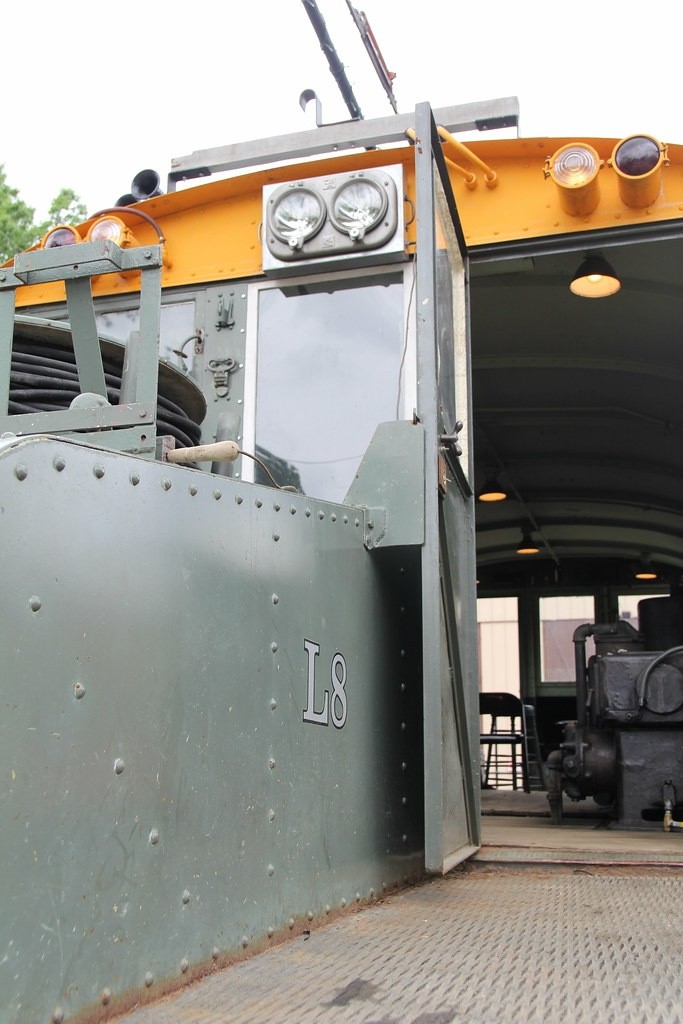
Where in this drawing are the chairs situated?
[479,692,531,795]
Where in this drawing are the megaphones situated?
[114,194,140,208]
[131,169,166,200]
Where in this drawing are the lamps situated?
[634,550,658,580]
[478,458,507,503]
[569,249,621,299]
[514,517,541,555]
